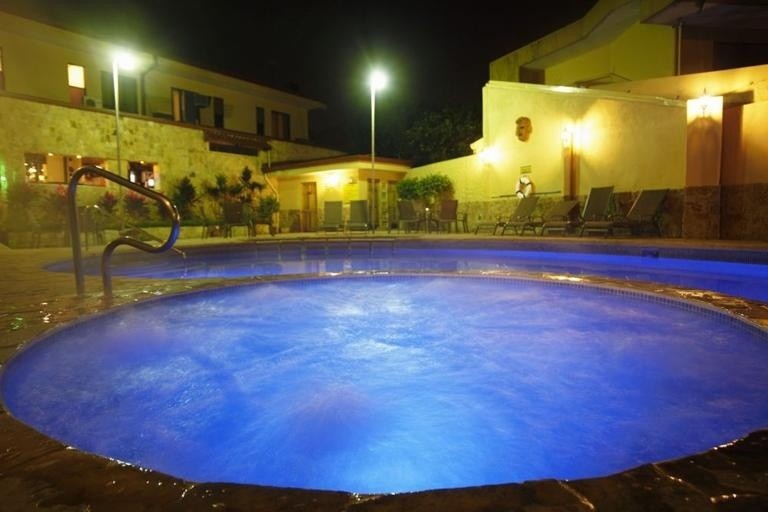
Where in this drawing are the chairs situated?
[493,196,540,235]
[396,199,426,235]
[200,205,228,239]
[250,206,274,236]
[579,188,670,239]
[521,199,580,237]
[346,200,375,237]
[457,202,471,235]
[543,186,613,237]
[320,200,342,235]
[428,199,460,233]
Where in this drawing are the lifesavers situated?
[515,177,533,197]
